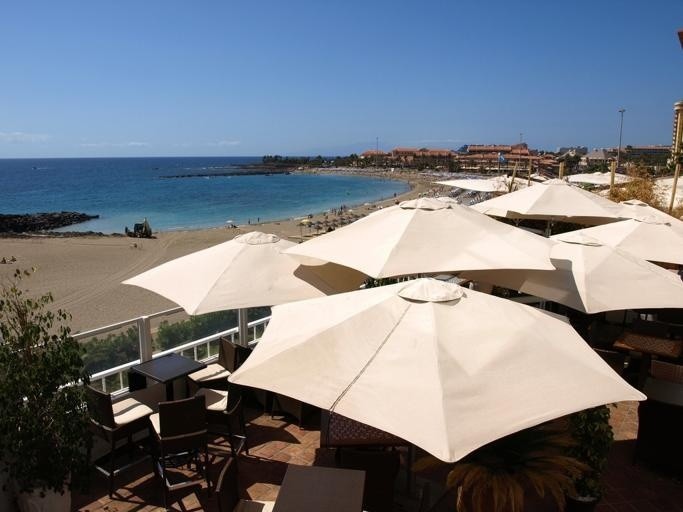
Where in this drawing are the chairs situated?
[79,300,457,512]
[568,308,683,392]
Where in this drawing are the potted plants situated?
[2,252,112,512]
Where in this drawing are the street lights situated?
[617,109,625,168]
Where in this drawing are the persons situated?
[0,257,7,264]
[11,255,16,262]
